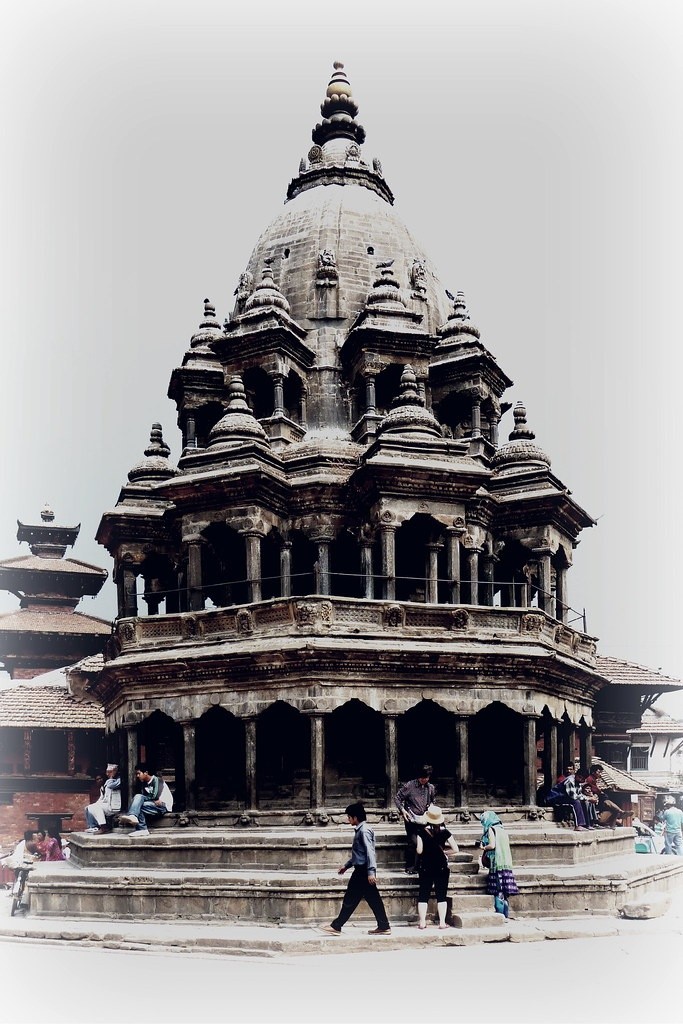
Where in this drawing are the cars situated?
[631,817,678,855]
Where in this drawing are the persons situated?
[652,798,683,856]
[393,765,437,875]
[17,827,71,862]
[318,803,392,935]
[415,806,459,928]
[540,759,634,830]
[479,811,519,923]
[84,763,121,835]
[117,762,173,837]
[90,773,104,804]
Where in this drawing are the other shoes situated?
[84,826,99,832]
[575,825,589,831]
[319,924,341,935]
[593,824,605,829]
[119,815,139,825]
[419,923,427,928]
[128,830,150,837]
[404,868,412,875]
[439,923,450,929]
[94,826,110,834]
[619,811,635,819]
[586,824,595,830]
[368,927,391,934]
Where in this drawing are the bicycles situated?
[0,849,42,916]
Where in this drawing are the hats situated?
[424,806,444,824]
[60,839,70,846]
[107,764,118,771]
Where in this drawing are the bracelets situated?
[484,845,487,850]
